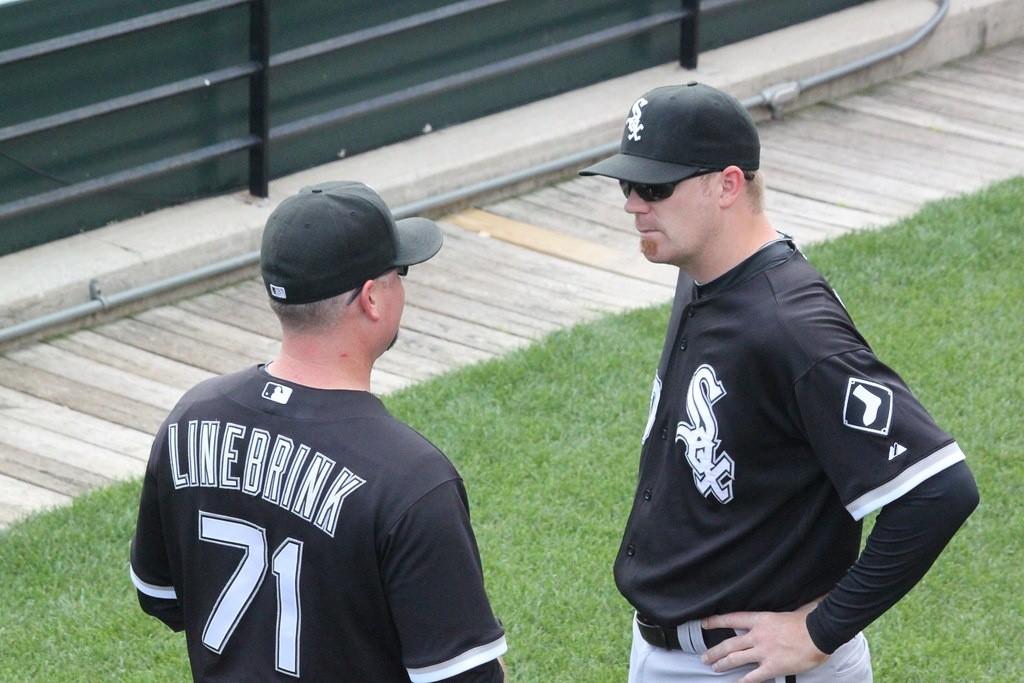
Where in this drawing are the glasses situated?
[347,264,408,305]
[620,168,754,202]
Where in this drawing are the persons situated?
[581,82,979,683]
[130,176,508,683]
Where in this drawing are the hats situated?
[260,180,443,304]
[578,81,760,184]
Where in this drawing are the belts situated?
[635,612,737,652]
